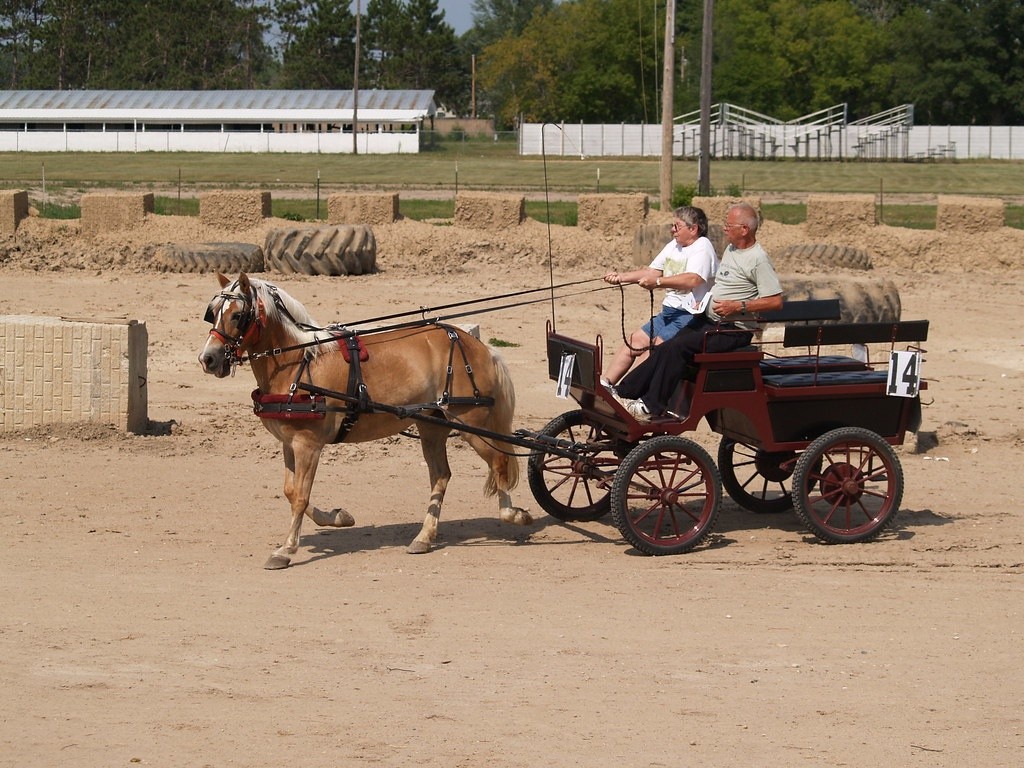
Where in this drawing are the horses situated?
[196,269,532,571]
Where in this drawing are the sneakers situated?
[612,393,653,423]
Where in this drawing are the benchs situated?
[690,317,767,362]
[760,300,875,376]
[762,320,930,398]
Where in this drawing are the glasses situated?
[671,222,685,231]
[723,221,743,229]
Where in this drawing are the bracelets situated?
[741,301,746,315]
[656,277,660,286]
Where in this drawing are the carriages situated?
[195,264,932,570]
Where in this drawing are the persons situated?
[609,204,784,423]
[600,206,719,387]
